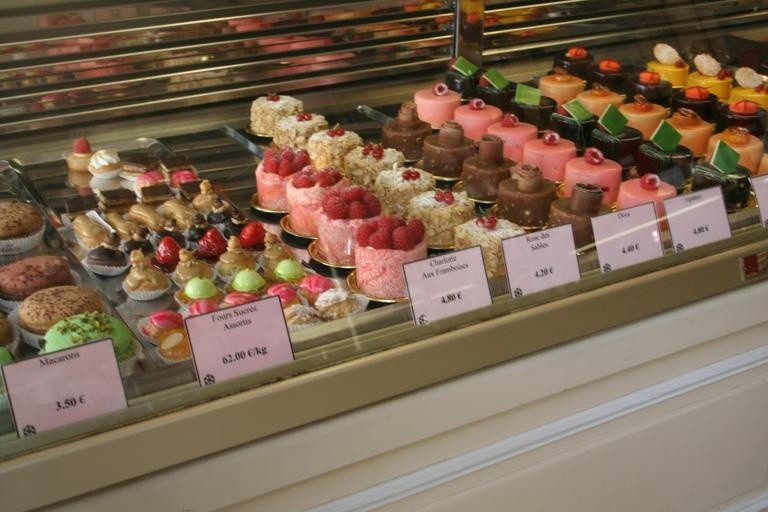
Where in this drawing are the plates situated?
[250,151,582,305]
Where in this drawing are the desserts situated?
[0,44,768,385]
